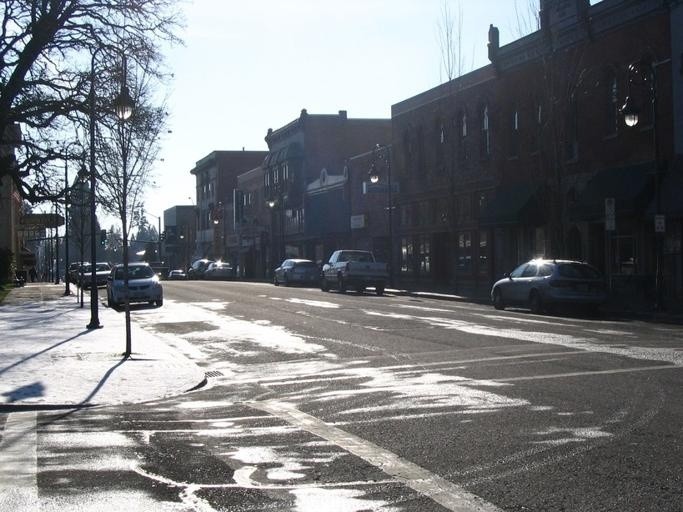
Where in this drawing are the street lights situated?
[177,230,186,263]
[264,192,287,259]
[621,53,666,313]
[87,45,134,330]
[62,142,91,298]
[208,200,227,260]
[368,142,395,288]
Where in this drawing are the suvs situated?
[188,258,216,280]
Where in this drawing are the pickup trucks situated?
[318,250,392,296]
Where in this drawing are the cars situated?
[106,262,163,311]
[67,261,114,290]
[491,259,609,317]
[205,263,233,280]
[168,269,186,280]
[272,257,319,290]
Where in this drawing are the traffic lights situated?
[100,230,105,245]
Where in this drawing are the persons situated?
[28,266,37,282]
